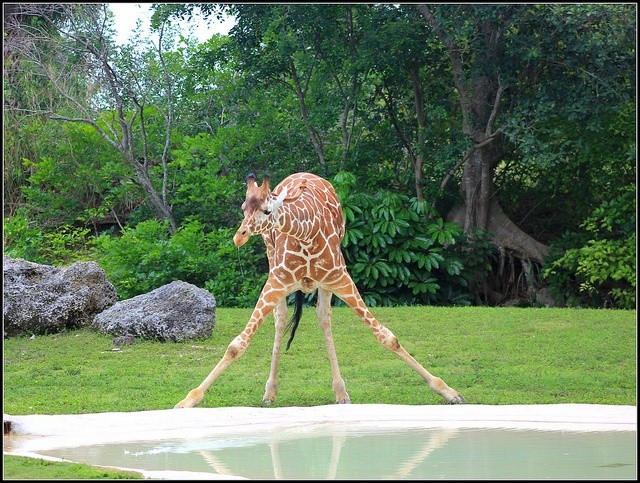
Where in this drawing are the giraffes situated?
[173,172,467,409]
